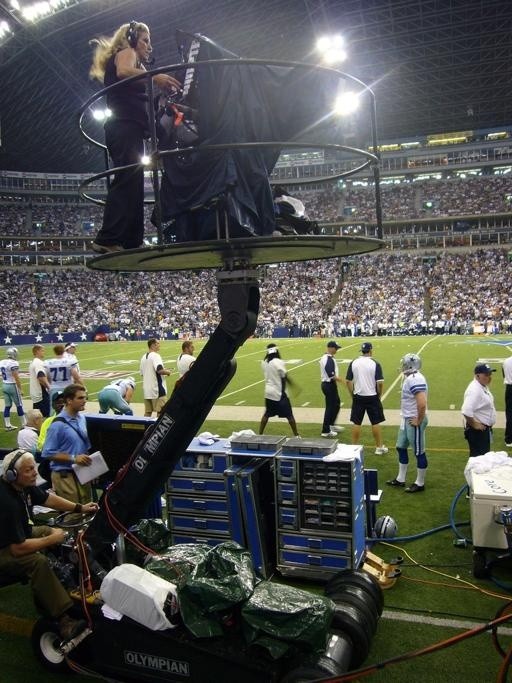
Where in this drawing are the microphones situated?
[145,57,156,65]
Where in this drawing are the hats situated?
[267,346,277,353]
[65,342,78,350]
[360,342,372,351]
[475,363,496,373]
[52,390,63,399]
[328,341,341,347]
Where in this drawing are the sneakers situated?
[330,425,344,431]
[405,483,424,492]
[60,617,85,640]
[321,431,338,436]
[375,444,388,454]
[386,478,406,486]
[5,424,18,430]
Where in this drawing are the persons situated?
[0,449,100,641]
[319,341,346,437]
[1,177,512,342]
[460,364,498,499]
[346,343,389,455]
[386,353,429,493]
[89,20,182,253]
[1,338,197,533]
[501,356,512,447]
[259,343,302,438]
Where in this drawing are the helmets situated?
[6,347,17,359]
[400,353,421,373]
[375,515,397,537]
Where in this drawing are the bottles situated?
[500,504,512,532]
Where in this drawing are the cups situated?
[452,539,467,548]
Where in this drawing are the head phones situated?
[5,449,28,482]
[126,22,138,47]
